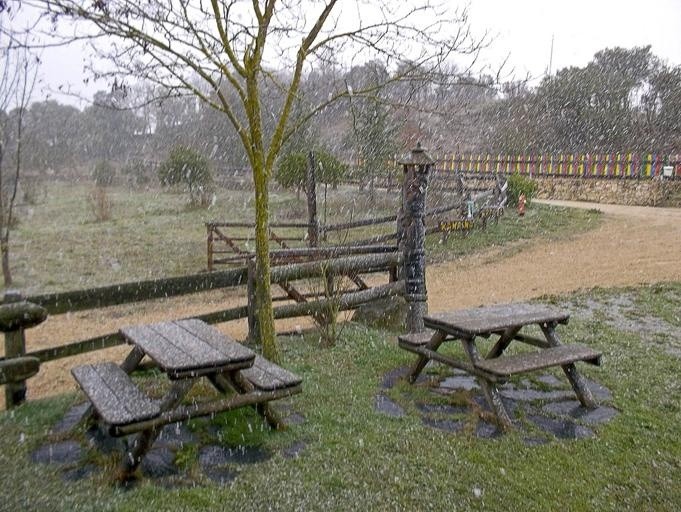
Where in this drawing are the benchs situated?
[474,344,602,378]
[398,331,457,346]
[71,361,160,425]
[240,353,302,391]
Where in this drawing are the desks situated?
[82,318,284,481]
[404,303,599,434]
